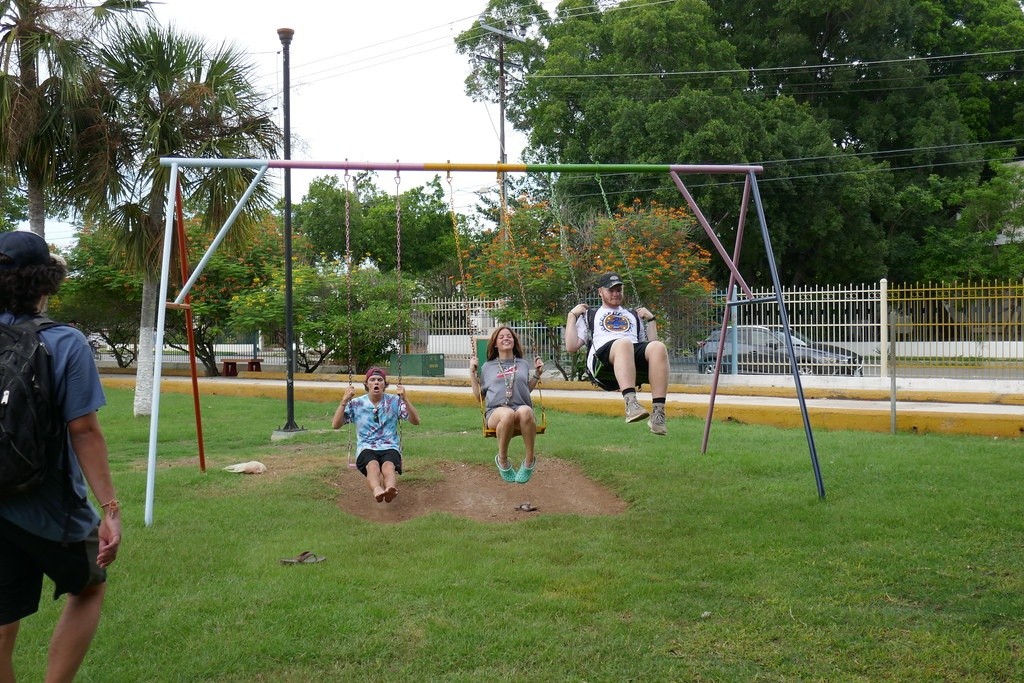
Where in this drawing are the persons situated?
[469,326,545,484]
[565,274,671,435]
[0,231,123,683]
[331,367,420,503]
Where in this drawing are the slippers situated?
[516,455,536,484]
[514,503,537,511]
[280,551,326,564]
[495,454,516,483]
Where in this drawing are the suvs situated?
[697,325,863,376]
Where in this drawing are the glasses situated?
[373,408,379,423]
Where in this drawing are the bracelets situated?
[340,402,347,406]
[100,500,120,518]
[570,311,577,318]
[533,375,540,380]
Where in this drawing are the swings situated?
[445,159,548,438]
[343,157,404,468]
[546,159,651,384]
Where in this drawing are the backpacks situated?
[0,320,69,499]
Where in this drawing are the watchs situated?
[648,316,656,322]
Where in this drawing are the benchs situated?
[220,359,263,377]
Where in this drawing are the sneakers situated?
[648,412,666,435]
[625,398,650,423]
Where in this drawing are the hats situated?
[597,272,624,289]
[365,367,386,392]
[0,231,66,272]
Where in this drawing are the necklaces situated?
[496,356,516,404]
